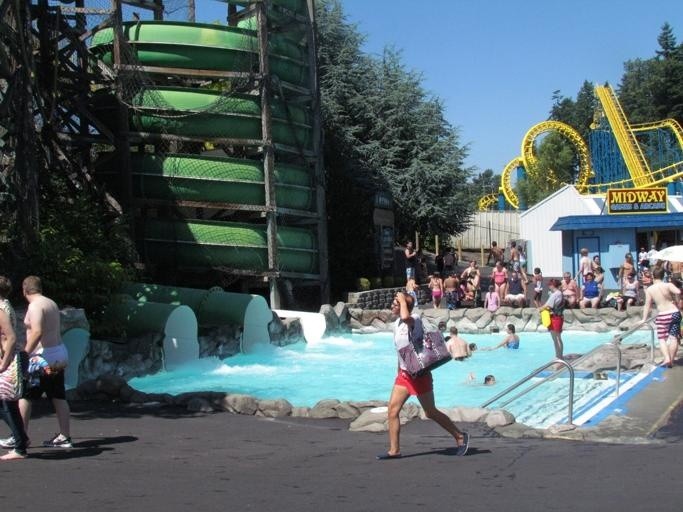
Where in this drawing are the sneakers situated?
[375,452,401,460]
[40,432,73,448]
[456,432,470,458]
[0,436,32,460]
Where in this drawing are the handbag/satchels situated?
[0,352,25,402]
[398,320,453,379]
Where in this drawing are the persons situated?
[0,275,30,462]
[374,291,469,463]
[0,274,75,451]
[403,238,682,389]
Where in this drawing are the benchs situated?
[458,299,530,307]
